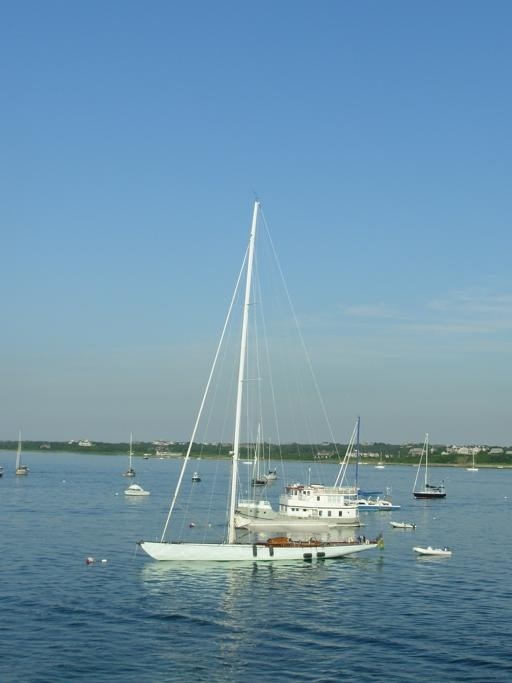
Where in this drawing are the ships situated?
[235,462,368,543]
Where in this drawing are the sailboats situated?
[251,416,400,511]
[135,199,384,561]
[122,432,135,476]
[466,446,480,471]
[139,558,359,671]
[15,428,31,474]
[411,431,447,499]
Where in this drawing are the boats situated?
[0,466,4,476]
[390,521,417,527]
[123,483,150,496]
[413,546,452,554]
[496,465,503,468]
[142,456,148,458]
[191,471,201,480]
